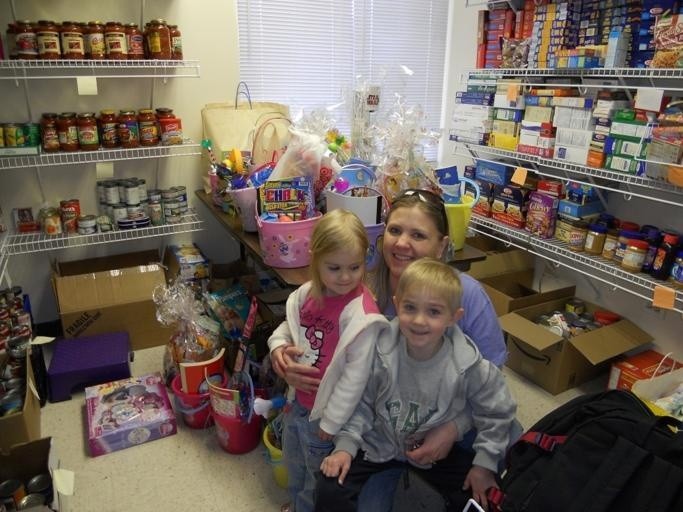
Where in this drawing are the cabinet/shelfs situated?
[447,0,682,317]
[1,60,203,292]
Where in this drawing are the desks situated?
[194,186,487,290]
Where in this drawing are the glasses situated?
[390,189,449,235]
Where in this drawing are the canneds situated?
[6,17,183,62]
[0,287,33,418]
[0,473,55,512]
[539,299,603,338]
[1,108,183,150]
[37,178,187,235]
[567,213,683,290]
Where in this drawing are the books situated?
[178,346,225,393]
[202,365,240,418]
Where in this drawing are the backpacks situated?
[486,389,682,511]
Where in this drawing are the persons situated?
[308,255,524,511]
[281,186,510,511]
[265,206,388,511]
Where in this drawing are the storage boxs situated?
[0,345,60,512]
[465,231,655,397]
[49,248,178,351]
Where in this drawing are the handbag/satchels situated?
[630,366,682,431]
[201,81,288,195]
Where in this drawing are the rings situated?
[428,459,437,465]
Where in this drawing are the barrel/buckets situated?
[259,422,290,490]
[208,406,263,455]
[442,176,481,253]
[210,152,391,272]
[171,371,228,428]
